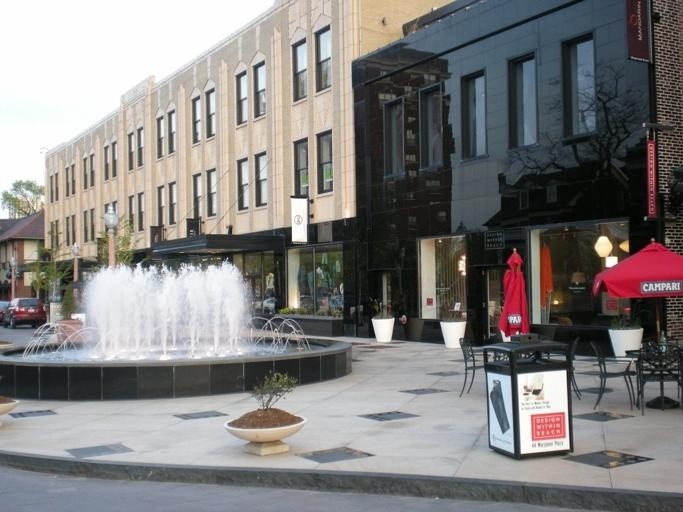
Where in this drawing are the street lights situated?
[9,255,16,303]
[104,197,122,269]
[71,240,81,314]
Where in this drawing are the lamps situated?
[571,271,586,287]
[594,223,629,268]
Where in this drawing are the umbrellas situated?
[497,247,530,337]
[539,241,554,324]
[590,238,683,398]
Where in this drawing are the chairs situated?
[458,335,683,414]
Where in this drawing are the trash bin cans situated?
[483,334,574,459]
[48,301,68,325]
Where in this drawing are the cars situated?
[0,302,10,321]
[249,292,344,318]
[2,297,48,329]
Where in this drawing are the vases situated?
[0,394,20,428]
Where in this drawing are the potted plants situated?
[608,309,644,361]
[223,369,308,456]
[440,301,466,349]
[370,302,395,342]
